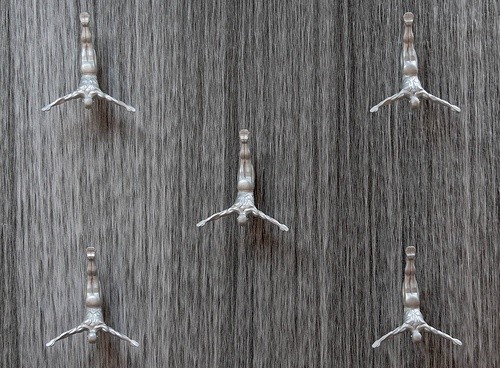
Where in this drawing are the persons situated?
[46,248,140,352]
[369,12,464,119]
[40,12,134,113]
[193,127,291,235]
[369,245,464,346]
